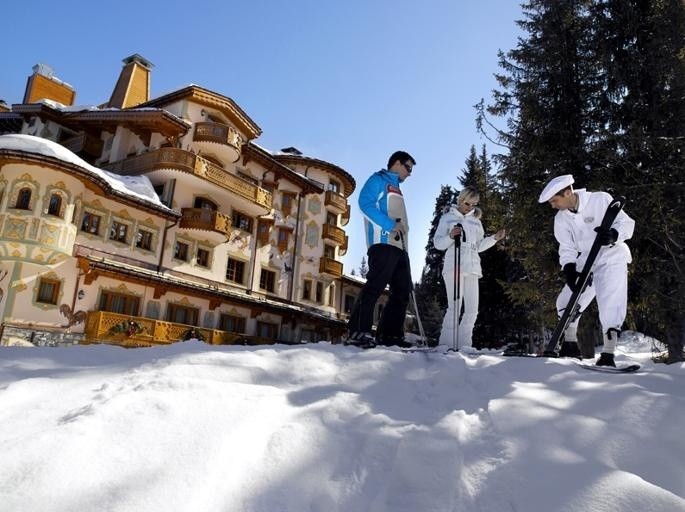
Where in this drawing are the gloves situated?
[594,227,616,246]
[564,263,585,293]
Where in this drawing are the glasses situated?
[403,162,412,173]
[464,200,477,207]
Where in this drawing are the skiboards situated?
[443,345,508,356]
[548,194,640,373]
[344,343,450,353]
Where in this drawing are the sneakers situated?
[596,353,615,366]
[376,334,412,347]
[342,331,377,348]
[558,342,583,359]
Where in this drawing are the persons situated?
[537,174,636,368]
[343,151,417,349]
[431,186,506,353]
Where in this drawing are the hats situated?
[538,175,575,203]
